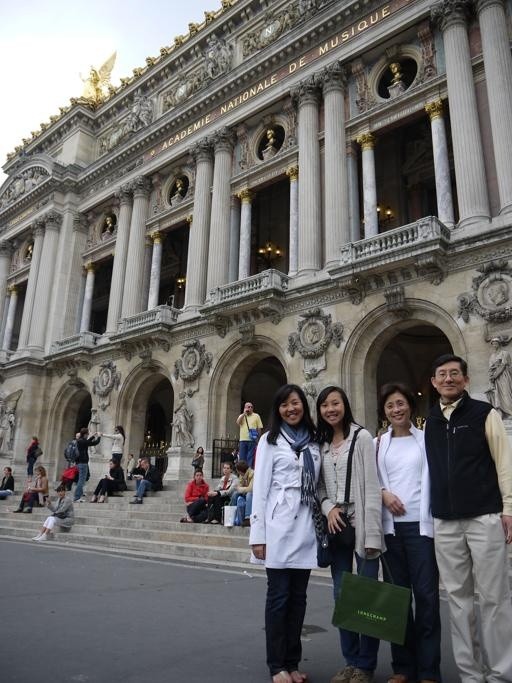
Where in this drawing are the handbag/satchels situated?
[318,511,355,567]
[33,448,42,458]
[54,512,66,518]
[249,429,259,440]
[23,493,31,501]
[223,506,238,527]
[192,458,200,466]
[332,570,411,646]
[60,467,79,479]
[206,503,221,522]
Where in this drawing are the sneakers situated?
[180,518,188,522]
[32,532,48,541]
[13,507,32,513]
[74,496,105,503]
[330,666,373,682]
[242,518,250,527]
[210,519,219,524]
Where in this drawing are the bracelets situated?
[241,414,244,416]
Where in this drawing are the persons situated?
[25,436,40,483]
[302,371,319,423]
[184,447,254,526]
[372,383,441,683]
[424,354,512,683]
[31,485,74,541]
[0,405,15,451]
[0,467,14,496]
[55,426,163,504]
[236,401,264,469]
[13,465,49,513]
[316,387,387,683]
[85,407,102,452]
[484,338,512,416]
[248,383,323,683]
[173,389,199,446]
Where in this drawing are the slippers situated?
[273,670,310,682]
[387,675,433,682]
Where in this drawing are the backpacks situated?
[64,439,80,460]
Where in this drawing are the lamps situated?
[258,186,282,268]
[361,203,395,234]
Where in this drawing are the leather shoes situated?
[134,493,147,497]
[129,499,142,504]
